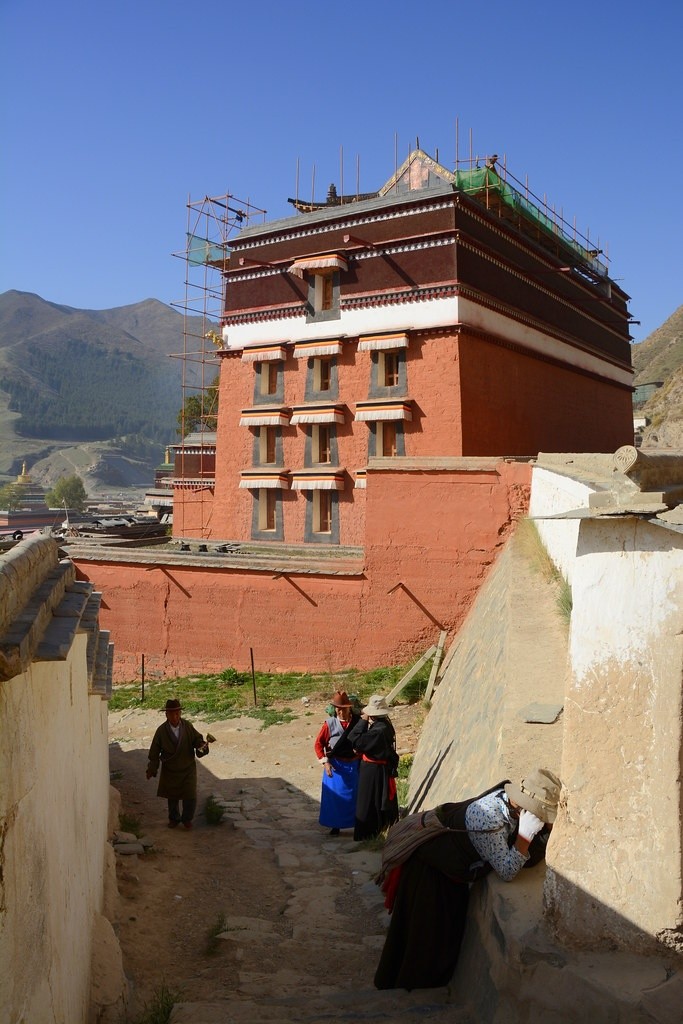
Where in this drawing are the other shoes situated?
[180,818,192,830]
[329,828,340,836]
[167,816,180,828]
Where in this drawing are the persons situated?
[145,699,209,830]
[315,691,362,835]
[374,769,562,992]
[347,695,400,841]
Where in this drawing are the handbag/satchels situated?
[374,809,446,882]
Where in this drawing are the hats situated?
[504,769,561,823]
[328,691,354,707]
[160,699,184,711]
[361,694,389,716]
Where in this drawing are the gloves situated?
[518,811,544,842]
